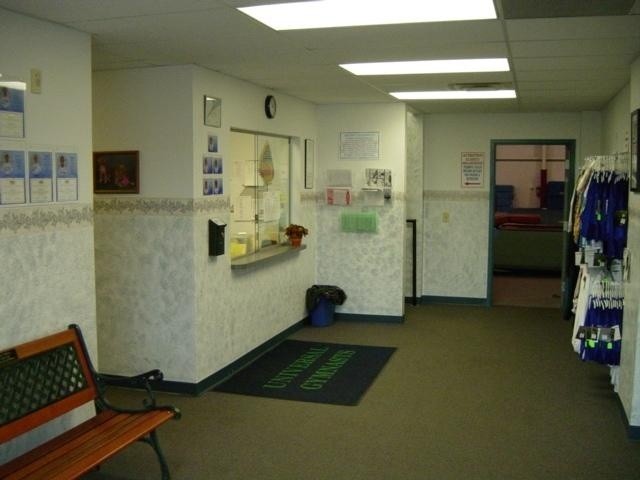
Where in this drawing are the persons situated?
[2,154,14,176]
[203,180,210,195]
[1,87,12,108]
[203,158,209,173]
[57,155,70,175]
[30,154,42,176]
[214,180,220,194]
[213,159,220,174]
[208,136,215,152]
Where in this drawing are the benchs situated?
[0,323,183,479]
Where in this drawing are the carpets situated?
[209,338,398,408]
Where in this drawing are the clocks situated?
[265,93,278,120]
[203,94,222,129]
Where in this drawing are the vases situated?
[289,239,303,247]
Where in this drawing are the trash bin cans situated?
[309,285,335,326]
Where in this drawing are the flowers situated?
[285,223,309,239]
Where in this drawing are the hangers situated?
[588,281,626,310]
[581,154,629,183]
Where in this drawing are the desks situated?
[491,208,562,279]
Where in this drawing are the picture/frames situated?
[629,107,639,196]
[92,149,143,197]
[303,137,317,190]
[325,188,353,207]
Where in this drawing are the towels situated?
[341,213,378,233]
